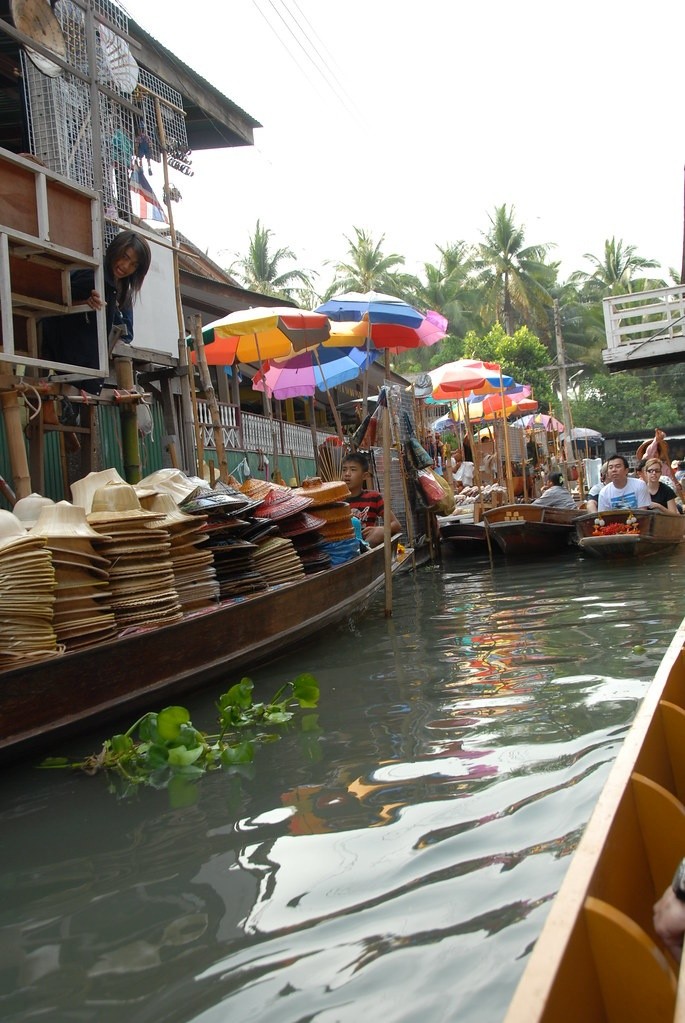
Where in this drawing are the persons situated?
[427,436,489,493]
[37,230,151,450]
[586,455,651,513]
[341,452,401,549]
[531,472,578,510]
[636,432,685,515]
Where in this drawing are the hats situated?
[636,439,669,462]
[670,460,679,468]
[0,468,356,670]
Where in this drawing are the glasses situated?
[646,469,661,473]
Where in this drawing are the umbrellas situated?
[187,306,331,483]
[252,291,448,482]
[556,427,606,459]
[510,413,564,461]
[420,357,539,464]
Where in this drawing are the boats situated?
[1,530,416,755]
[426,372,588,561]
[505,618,685,1023]
[571,507,685,562]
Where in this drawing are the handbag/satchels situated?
[403,412,455,517]
[348,389,388,451]
